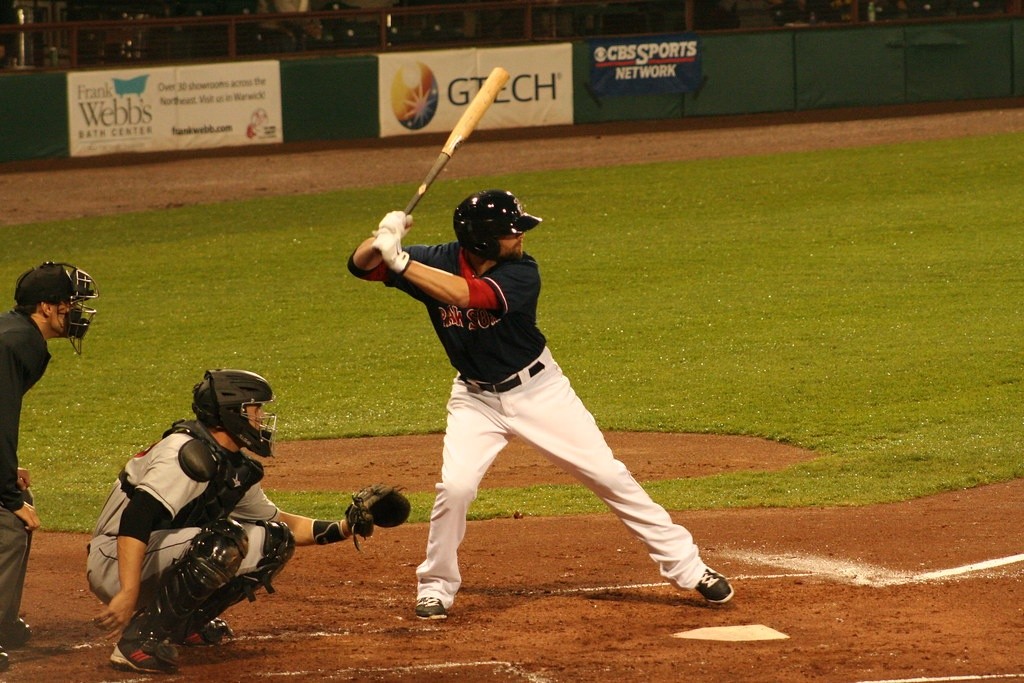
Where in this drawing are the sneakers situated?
[415,597,448,619]
[110,607,182,673]
[169,621,207,645]
[694,567,735,603]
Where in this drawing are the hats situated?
[14,261,95,303]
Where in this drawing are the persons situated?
[347,189,735,620]
[87,368,411,672]
[0,261,98,672]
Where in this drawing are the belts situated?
[87,534,113,555]
[459,361,544,393]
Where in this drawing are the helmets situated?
[453,189,542,260]
[191,368,276,458]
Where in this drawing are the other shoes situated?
[0,646,8,666]
[0,619,33,645]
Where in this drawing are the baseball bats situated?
[372,66,512,258]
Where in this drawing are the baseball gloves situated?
[344,479,414,552]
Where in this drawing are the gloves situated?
[371,210,412,273]
